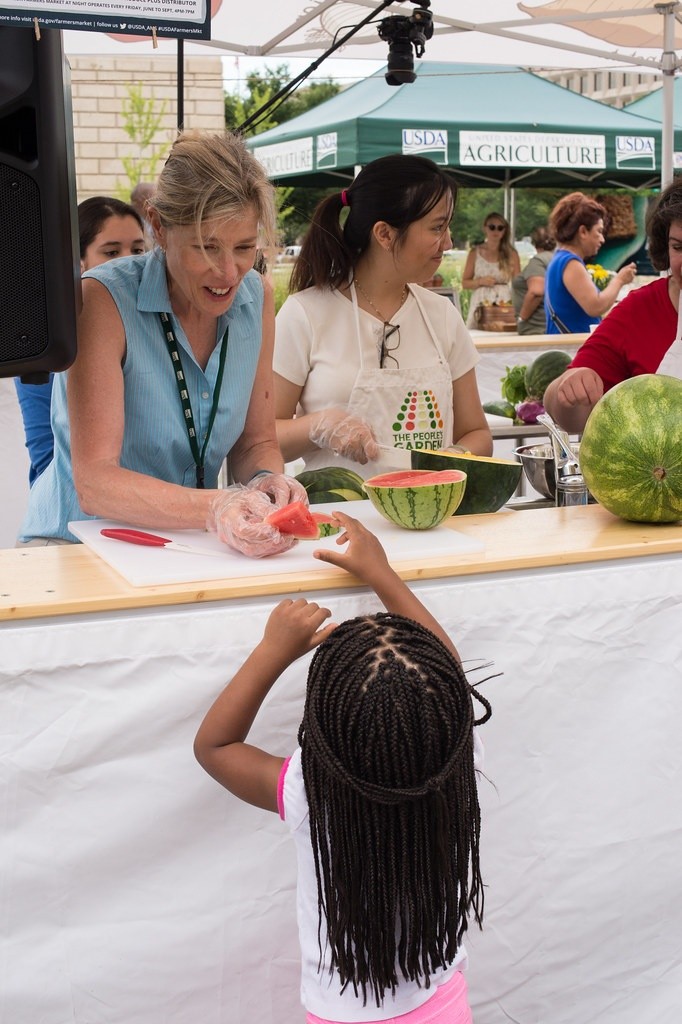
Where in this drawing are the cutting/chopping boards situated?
[68,500,487,587]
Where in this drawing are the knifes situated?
[100,528,238,561]
[364,445,411,468]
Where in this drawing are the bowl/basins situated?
[513,442,594,501]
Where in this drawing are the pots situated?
[474,305,516,330]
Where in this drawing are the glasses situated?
[486,224,507,231]
[380,322,400,369]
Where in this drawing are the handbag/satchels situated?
[474,303,517,332]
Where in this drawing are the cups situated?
[551,433,587,507]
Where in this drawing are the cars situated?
[276,245,302,263]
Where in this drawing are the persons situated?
[543,192,637,333]
[543,182,682,433]
[462,212,521,326]
[130,183,155,247]
[14,198,145,485]
[511,227,556,334]
[273,154,494,481]
[16,134,311,557]
[193,510,493,1024]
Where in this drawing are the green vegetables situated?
[500,365,528,404]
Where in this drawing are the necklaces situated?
[354,278,406,320]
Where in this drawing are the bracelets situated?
[518,316,523,321]
[252,470,273,478]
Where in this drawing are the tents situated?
[104,1,682,276]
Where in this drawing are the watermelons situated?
[482,400,516,419]
[411,448,522,516]
[364,469,467,530]
[309,512,341,537]
[294,466,368,504]
[579,374,682,522]
[264,500,321,541]
[524,351,572,400]
